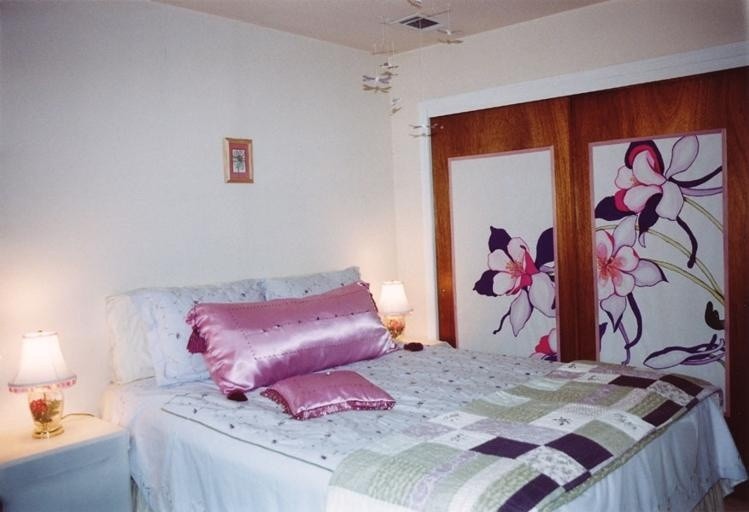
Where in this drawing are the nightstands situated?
[1,415,130,512]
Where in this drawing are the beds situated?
[100,346,724,512]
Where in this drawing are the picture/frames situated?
[225,138,254,184]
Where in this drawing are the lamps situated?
[8,330,77,440]
[376,280,413,345]
